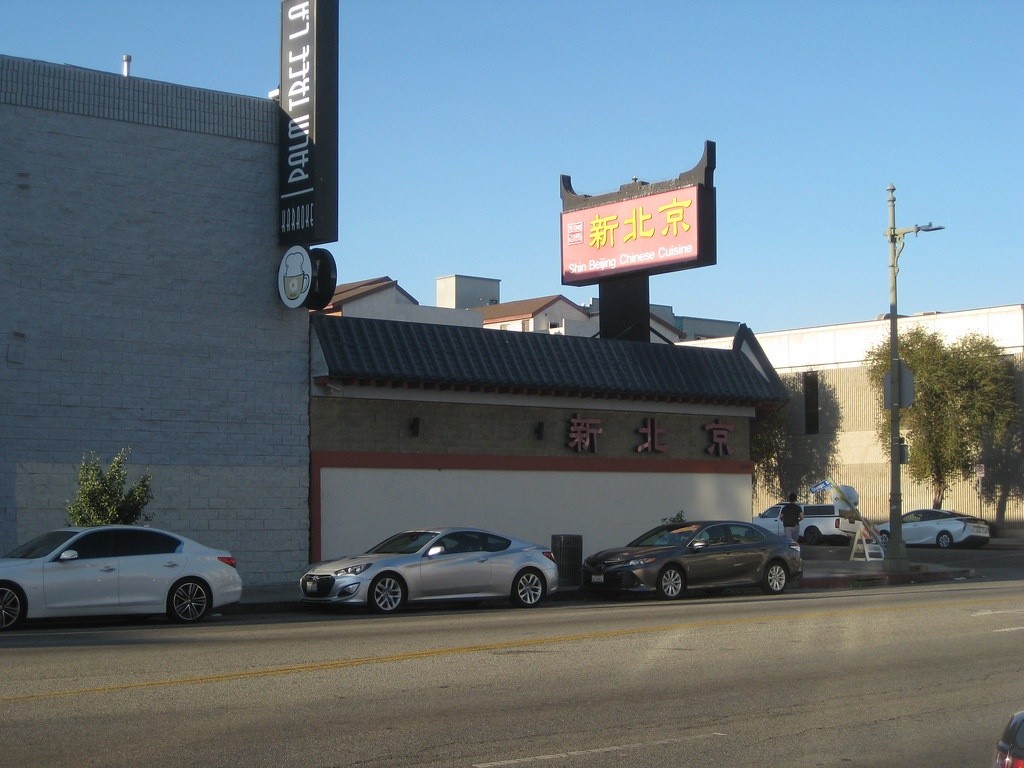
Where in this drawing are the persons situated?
[780,493,804,542]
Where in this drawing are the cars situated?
[579,520,805,601]
[0,524,244,632]
[872,508,990,550]
[298,526,560,615]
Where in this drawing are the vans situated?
[753,501,871,547]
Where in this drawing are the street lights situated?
[883,180,946,576]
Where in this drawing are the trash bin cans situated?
[551,534,583,587]
